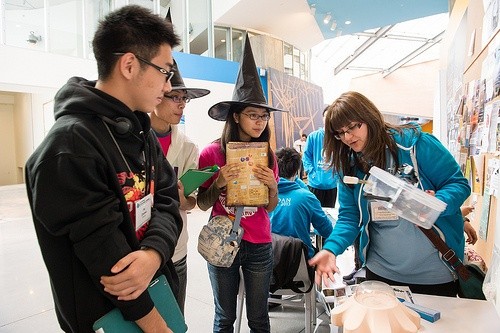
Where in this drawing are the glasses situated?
[164,94,192,104]
[333,121,364,140]
[111,52,175,83]
[240,111,271,122]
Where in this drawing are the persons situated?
[293,134,307,175]
[24,4,183,333]
[302,106,340,208]
[459,205,478,245]
[148,7,210,319]
[267,147,334,267]
[196,32,278,333]
[308,91,471,297]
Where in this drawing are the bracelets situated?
[463,218,470,222]
[269,189,279,198]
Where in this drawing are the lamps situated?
[310,5,337,30]
[28,30,42,46]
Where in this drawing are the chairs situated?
[231,229,325,333]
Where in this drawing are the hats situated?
[169,57,211,100]
[208,33,290,121]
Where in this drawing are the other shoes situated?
[267,296,283,309]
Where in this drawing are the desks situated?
[343,293,500,333]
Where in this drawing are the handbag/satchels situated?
[197,214,244,269]
[456,243,489,301]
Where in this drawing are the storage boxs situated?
[361,166,448,230]
